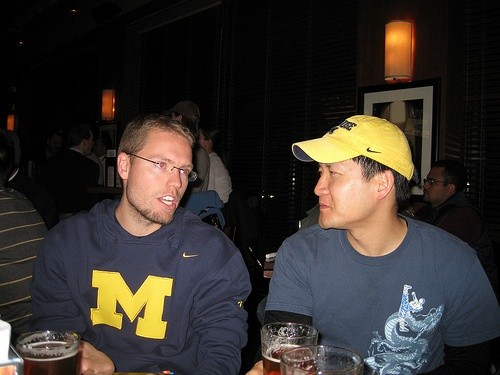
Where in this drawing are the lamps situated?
[384,17,416,81]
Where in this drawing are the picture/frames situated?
[357,77,439,203]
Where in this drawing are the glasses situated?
[126,152,198,182]
[424,178,454,185]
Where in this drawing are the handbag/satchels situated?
[185,189,226,231]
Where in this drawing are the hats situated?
[291,114,414,181]
[170,100,201,122]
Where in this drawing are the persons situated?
[0,124,112,346]
[244,115,500,375]
[32,114,253,375]
[163,101,500,305]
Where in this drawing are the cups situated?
[280,345,364,375]
[260,322,319,375]
[16,329,81,375]
[0,320,12,362]
